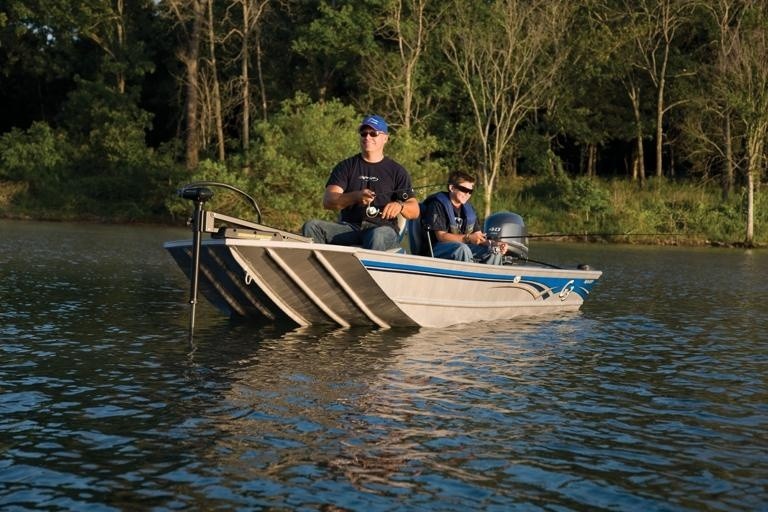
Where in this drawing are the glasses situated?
[360,131,384,138]
[455,184,474,194]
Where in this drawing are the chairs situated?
[388,213,407,253]
[409,202,442,256]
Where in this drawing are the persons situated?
[417,167,510,268]
[300,111,423,254]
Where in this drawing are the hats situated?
[357,115,389,134]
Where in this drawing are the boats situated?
[162,182,603,331]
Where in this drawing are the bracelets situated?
[395,199,405,215]
[465,232,472,244]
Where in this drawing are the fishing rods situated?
[364,181,466,219]
[500,254,563,269]
[484,233,704,243]
